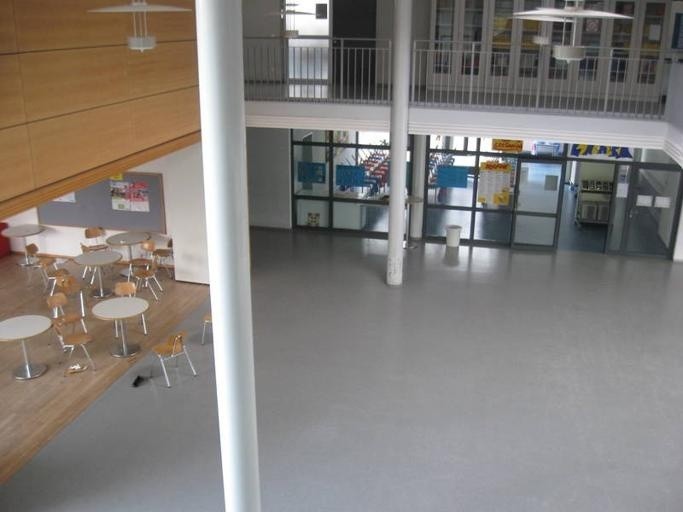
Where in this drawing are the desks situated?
[0,315,53,380]
[1,224,45,266]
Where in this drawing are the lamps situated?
[264,0,315,39]
[88,0,192,50]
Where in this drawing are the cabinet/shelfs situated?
[578,192,611,223]
[425,0,671,104]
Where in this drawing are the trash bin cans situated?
[445,224,462,248]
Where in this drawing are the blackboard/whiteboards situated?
[37,172,167,235]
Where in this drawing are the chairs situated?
[25,227,212,388]
